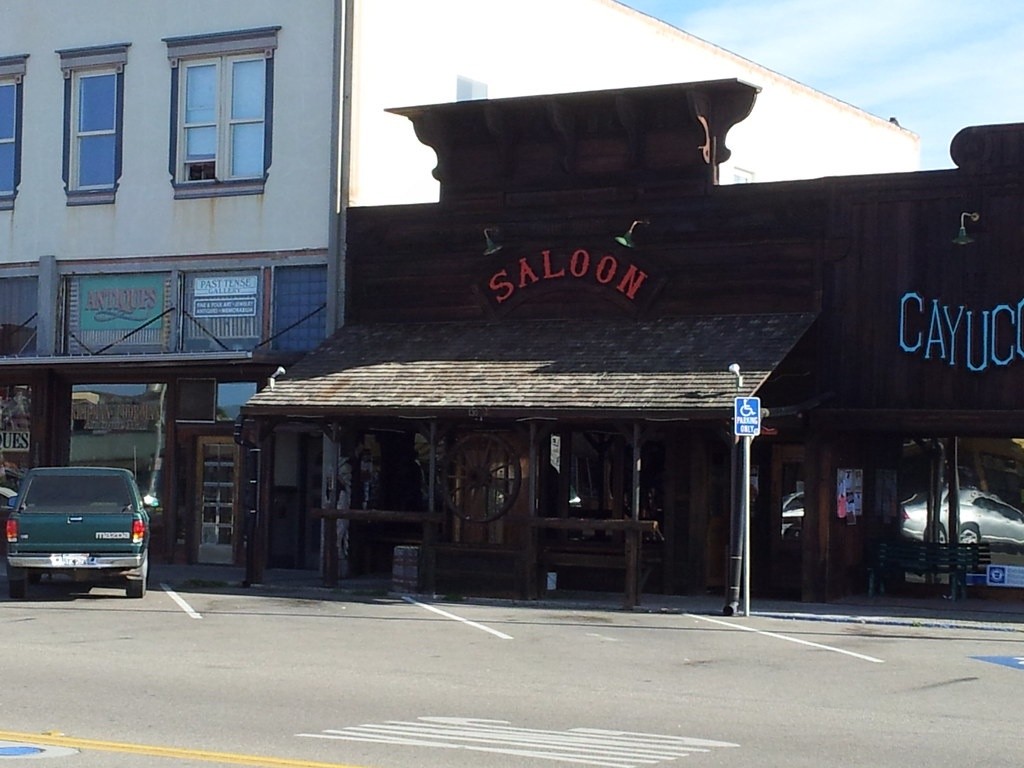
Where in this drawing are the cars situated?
[779,489,807,540]
[897,487,1024,548]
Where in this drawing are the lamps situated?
[481,227,503,255]
[951,211,980,244]
[616,219,651,247]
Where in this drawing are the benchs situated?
[866,539,993,606]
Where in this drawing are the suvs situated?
[5,467,150,599]
